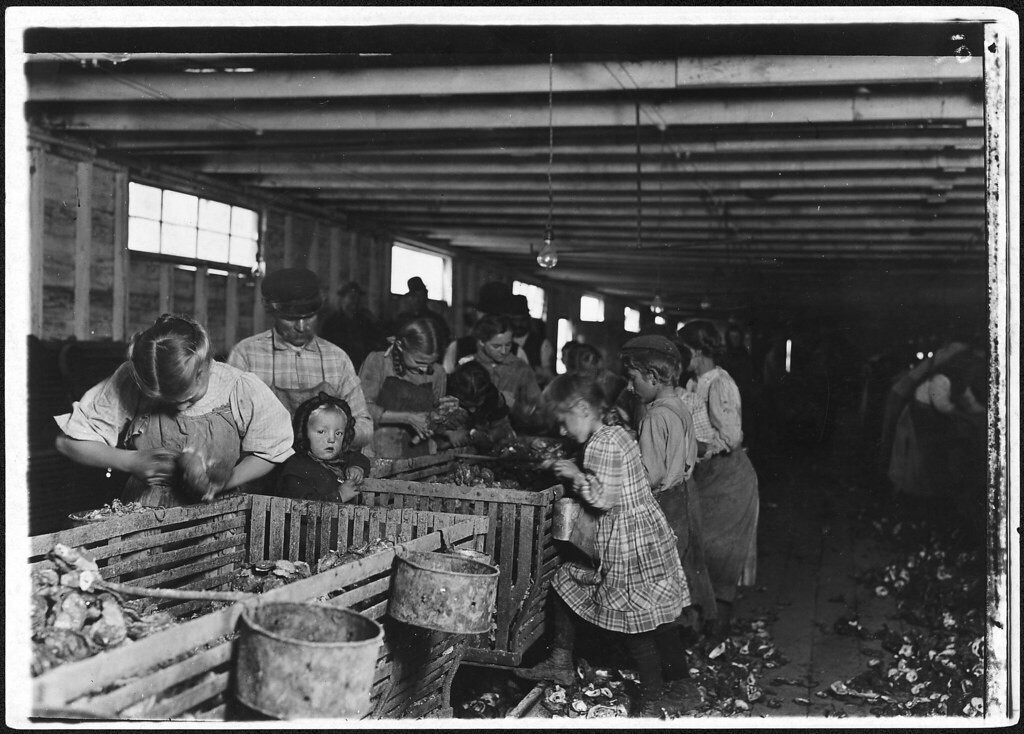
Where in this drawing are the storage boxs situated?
[31,493,490,720]
[356,443,564,667]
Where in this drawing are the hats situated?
[473,281,512,312]
[407,277,424,288]
[508,295,529,314]
[261,268,324,317]
[622,335,685,362]
[337,282,365,296]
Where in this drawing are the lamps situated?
[535,51,561,271]
[651,133,666,315]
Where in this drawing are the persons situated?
[610,334,719,713]
[397,277,556,432]
[319,281,377,377]
[435,360,509,448]
[227,268,373,494]
[358,318,459,458]
[676,317,757,640]
[276,391,370,502]
[52,313,295,589]
[513,370,693,720]
[542,342,626,419]
[881,342,983,502]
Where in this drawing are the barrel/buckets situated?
[389,550,501,636]
[232,600,386,720]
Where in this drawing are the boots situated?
[514,647,575,687]
[631,647,666,701]
